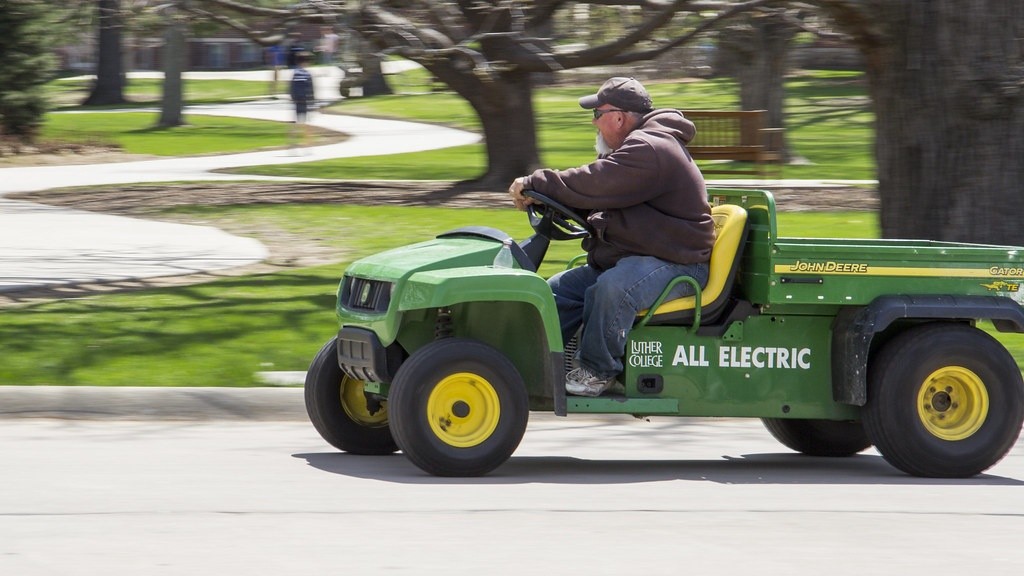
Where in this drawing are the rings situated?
[510,189,514,192]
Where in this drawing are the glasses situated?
[594,108,628,118]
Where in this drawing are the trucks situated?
[304,186,1024,477]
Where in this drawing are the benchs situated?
[682,110,787,177]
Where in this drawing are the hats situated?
[579,77,651,112]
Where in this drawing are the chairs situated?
[633,203,750,328]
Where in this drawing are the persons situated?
[509,76,715,396]
[287,24,339,124]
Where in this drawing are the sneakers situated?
[566,368,616,398]
